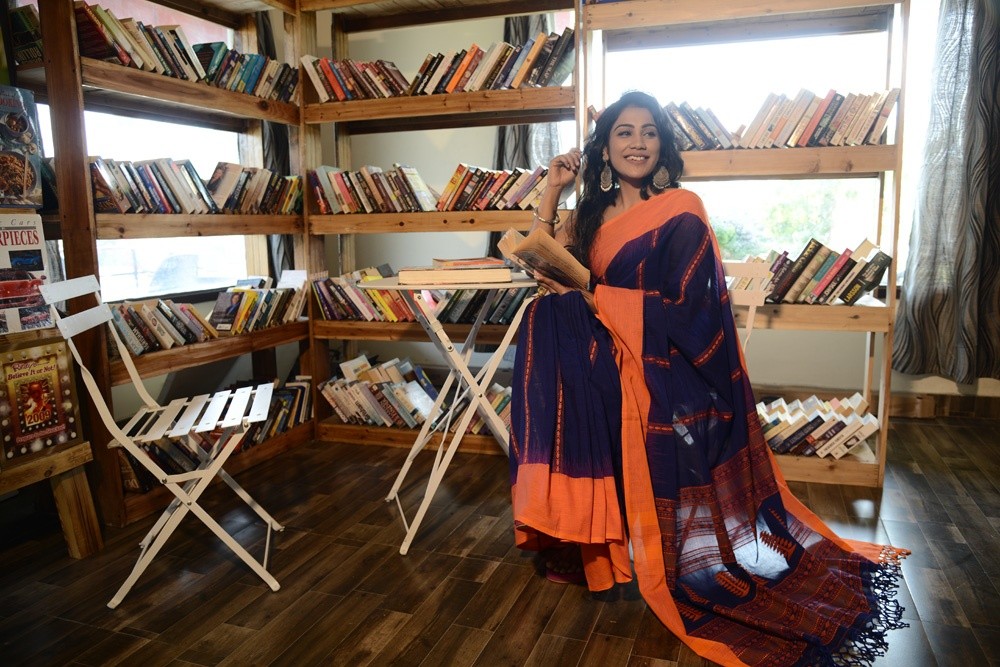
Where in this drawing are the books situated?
[498,227,593,291]
[41,155,303,215]
[725,236,893,307]
[115,376,314,492]
[299,27,577,104]
[0,339,83,470]
[0,212,57,334]
[587,88,901,150]
[756,391,880,460]
[317,355,512,435]
[312,256,545,325]
[311,161,578,216]
[0,86,46,210]
[103,269,309,358]
[2,0,300,105]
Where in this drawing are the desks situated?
[354,270,555,564]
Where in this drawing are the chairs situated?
[31,272,288,608]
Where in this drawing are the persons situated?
[509,90,718,584]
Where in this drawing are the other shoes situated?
[545,548,587,584]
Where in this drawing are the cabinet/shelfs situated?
[12,0,312,529]
[308,0,589,452]
[580,0,912,519]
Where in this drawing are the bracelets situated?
[532,206,562,224]
